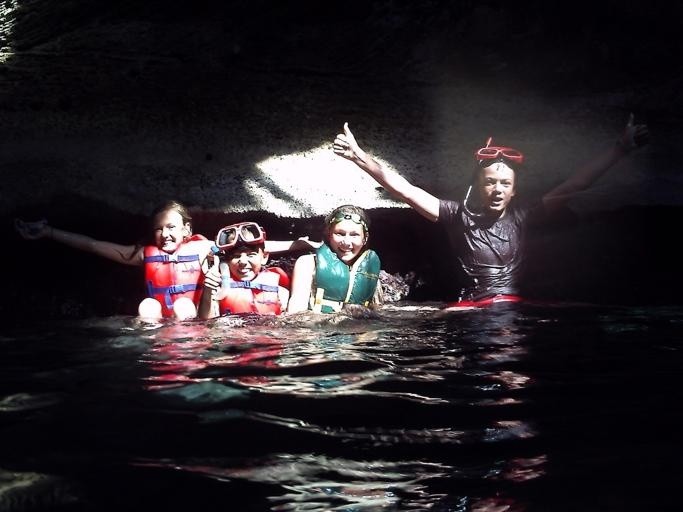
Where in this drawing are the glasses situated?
[215,222,266,250]
[330,213,369,232]
[475,146,523,164]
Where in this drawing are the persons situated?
[191,218,294,324]
[11,198,325,326]
[332,112,650,305]
[282,204,382,324]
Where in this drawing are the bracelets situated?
[44,226,66,251]
